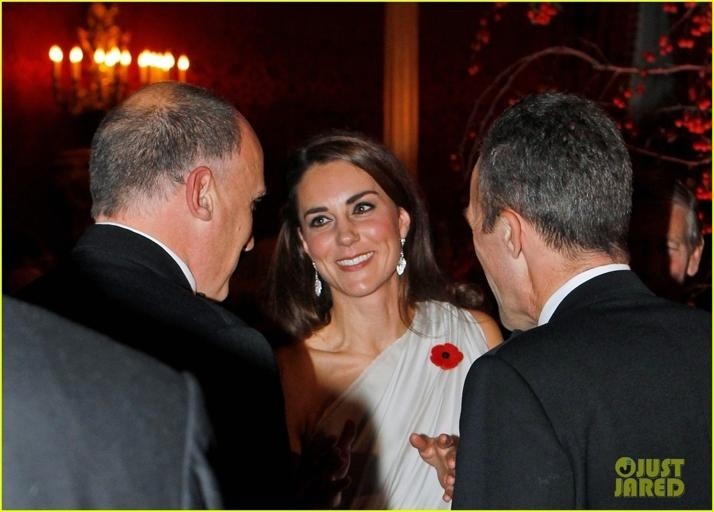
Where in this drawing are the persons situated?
[627,165,704,303]
[19,82,287,512]
[451,91,710,508]
[269,136,503,512]
